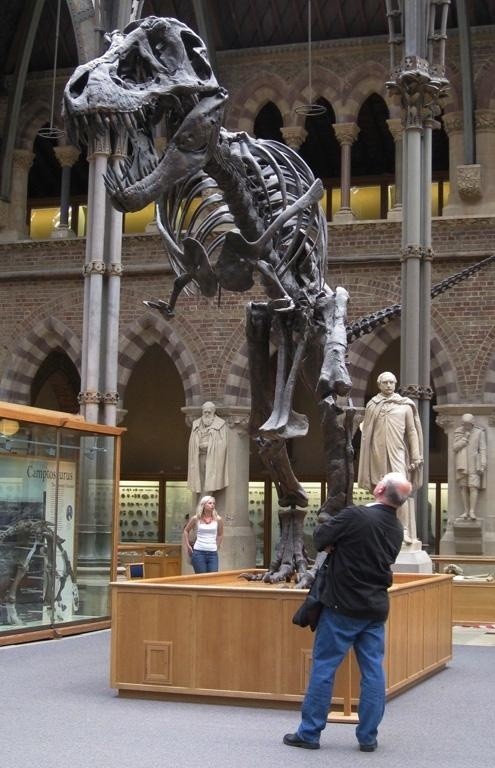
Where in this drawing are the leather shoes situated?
[360,739,378,751]
[283,733,320,750]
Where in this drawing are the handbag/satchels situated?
[292,596,322,632]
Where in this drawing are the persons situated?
[183,494,223,573]
[67,505,72,520]
[187,400,229,494]
[451,412,486,520]
[280,472,413,752]
[358,370,426,545]
